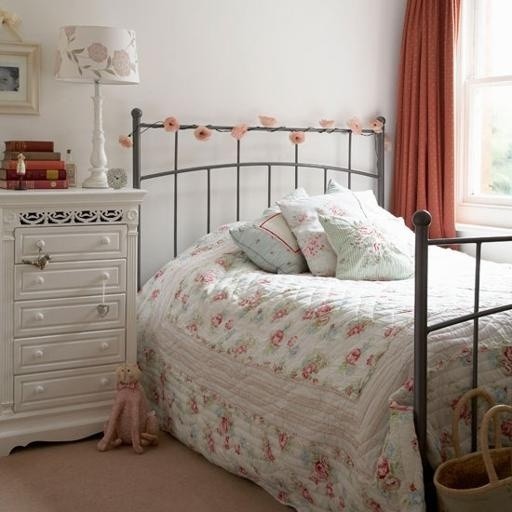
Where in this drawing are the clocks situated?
[107,168,128,189]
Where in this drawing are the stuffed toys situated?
[98,363,158,455]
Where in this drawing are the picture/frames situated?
[0,40,41,114]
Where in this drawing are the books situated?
[0,140,68,190]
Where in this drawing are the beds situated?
[131,108,512,512]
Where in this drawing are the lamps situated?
[52,23,139,189]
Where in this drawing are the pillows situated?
[228,178,418,281]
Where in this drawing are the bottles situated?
[64,148,77,187]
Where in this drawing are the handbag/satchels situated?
[432,389,511,512]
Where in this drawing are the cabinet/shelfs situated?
[0,187,148,458]
